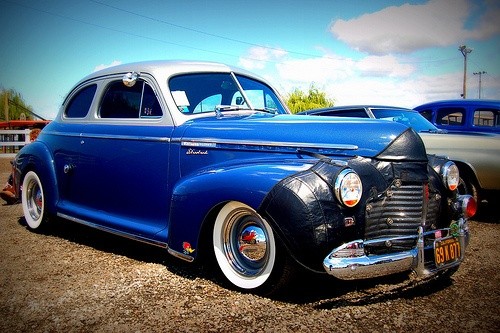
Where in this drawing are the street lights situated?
[459,45,472,100]
[473,70,486,99]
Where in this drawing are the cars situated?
[7,60,477,297]
[412,100,500,138]
[294,105,500,219]
